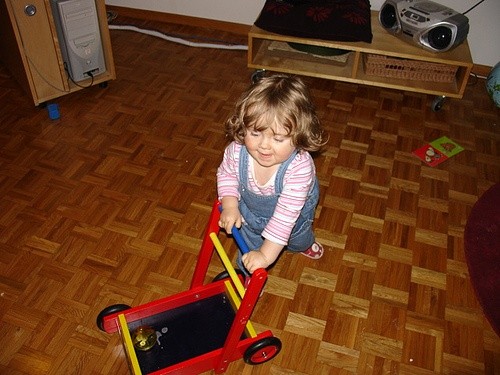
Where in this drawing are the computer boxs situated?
[57,0,106,82]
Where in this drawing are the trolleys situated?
[94,200,282,375]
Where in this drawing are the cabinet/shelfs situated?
[0,0,117,108]
[247,8,473,109]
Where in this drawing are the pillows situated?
[253,0,372,44]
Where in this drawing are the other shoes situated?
[245,276,264,297]
[300,241,325,259]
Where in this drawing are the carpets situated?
[464,182,500,338]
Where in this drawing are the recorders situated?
[378,0,485,52]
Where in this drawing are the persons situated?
[216,75,330,296]
